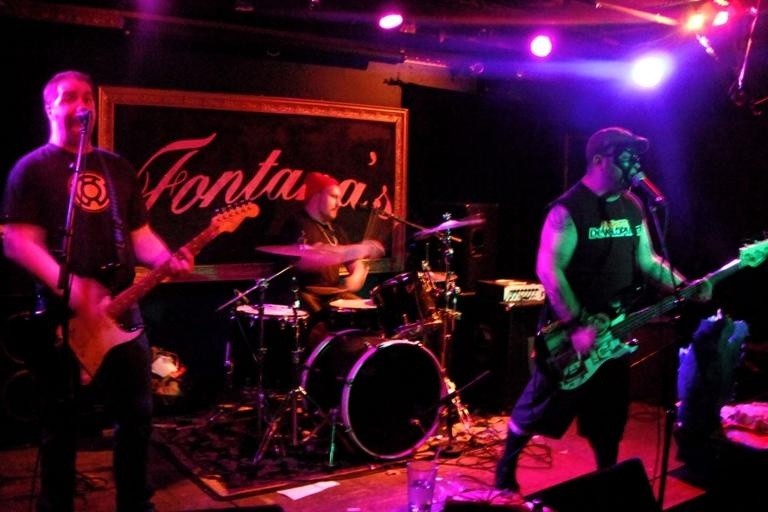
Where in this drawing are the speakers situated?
[508,456,664,511]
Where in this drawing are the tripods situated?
[215,271,358,465]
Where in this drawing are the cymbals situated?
[254,246,346,261]
[414,221,484,235]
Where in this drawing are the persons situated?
[260,170,386,351]
[493,126,714,494]
[3,70,198,511]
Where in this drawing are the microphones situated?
[76,108,92,123]
[628,167,667,208]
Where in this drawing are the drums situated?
[233,303,312,392]
[371,273,443,341]
[299,327,448,461]
[328,296,384,337]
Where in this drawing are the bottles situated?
[406,462,436,512]
[532,498,543,511]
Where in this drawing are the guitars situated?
[54,196,261,383]
[534,239,768,394]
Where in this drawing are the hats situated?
[305,172,340,205]
[586,127,649,158]
